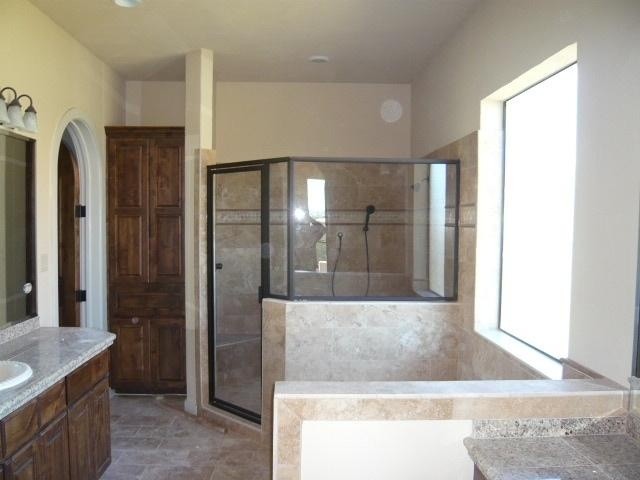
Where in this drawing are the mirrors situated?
[1,128,41,344]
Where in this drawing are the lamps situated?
[0,87,38,131]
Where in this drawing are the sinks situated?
[0,361,32,390]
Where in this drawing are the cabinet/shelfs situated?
[65,349,112,480]
[104,125,186,394]
[0,376,70,480]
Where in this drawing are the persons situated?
[290,208,326,273]
